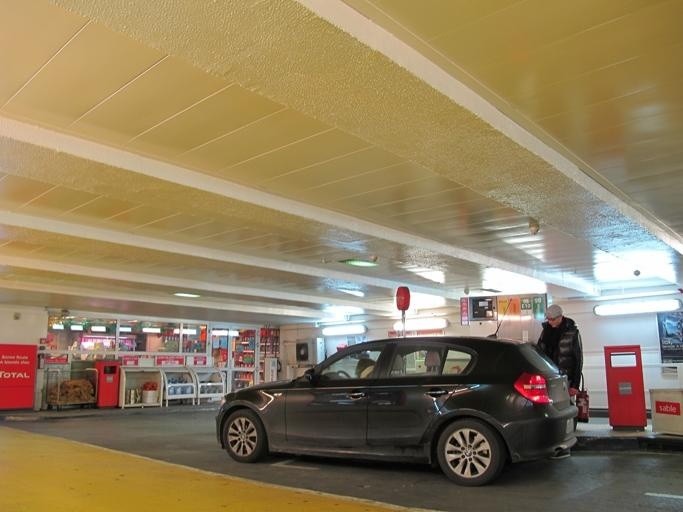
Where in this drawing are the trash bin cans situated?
[604,345,647,430]
[93,360,122,409]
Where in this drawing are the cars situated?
[216,297,579,486]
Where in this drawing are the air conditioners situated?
[295,337,326,369]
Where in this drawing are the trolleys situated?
[45,367,99,412]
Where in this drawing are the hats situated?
[546,304,563,320]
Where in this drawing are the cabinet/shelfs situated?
[230,330,279,392]
[118,366,225,409]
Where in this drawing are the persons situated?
[535,304,583,460]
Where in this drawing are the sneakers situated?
[548,447,571,460]
